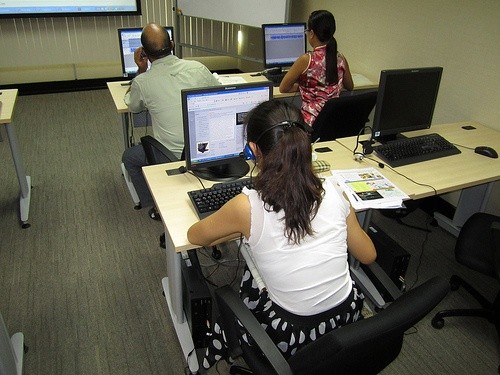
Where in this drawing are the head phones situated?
[139,40,174,57]
[244,122,305,161]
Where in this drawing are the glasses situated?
[303,29,311,34]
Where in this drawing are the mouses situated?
[475,147,499,157]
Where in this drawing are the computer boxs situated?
[180,250,213,348]
[366,222,411,302]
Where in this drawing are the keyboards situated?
[375,133,461,167]
[189,174,261,219]
[265,71,289,87]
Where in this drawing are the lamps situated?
[239,25,242,32]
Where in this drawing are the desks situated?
[0,70,500,375]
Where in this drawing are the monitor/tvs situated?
[262,23,308,72]
[373,66,444,144]
[117,26,175,79]
[181,80,275,182]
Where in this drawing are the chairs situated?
[140,134,185,222]
[431,211,500,329]
[309,92,377,144]
[214,260,455,375]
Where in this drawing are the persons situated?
[280,9,355,132]
[185,101,376,375]
[121,22,221,221]
